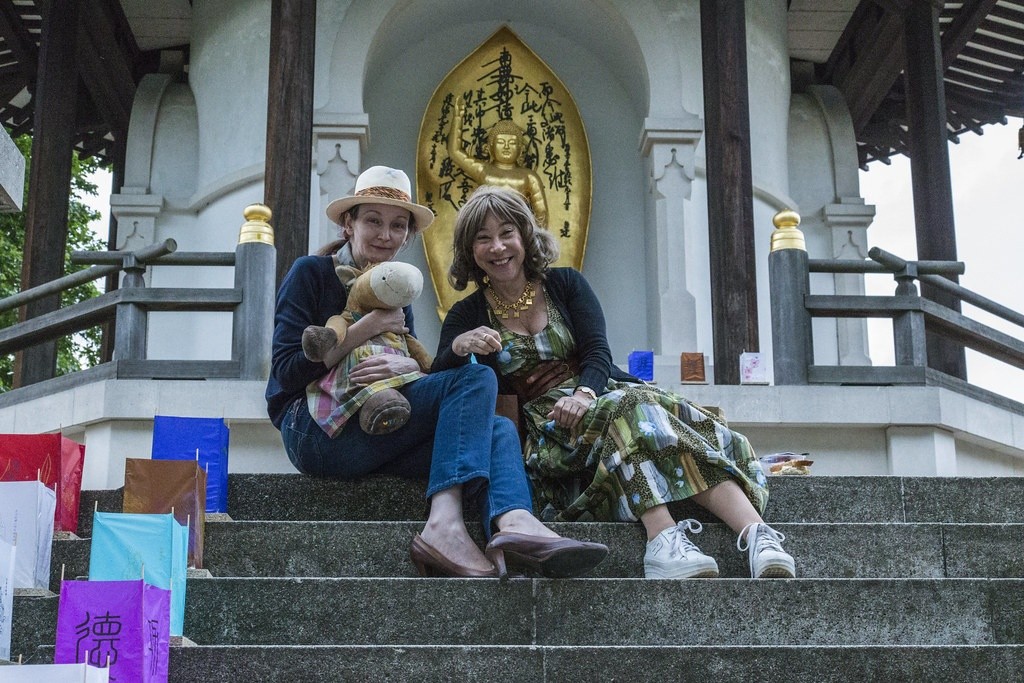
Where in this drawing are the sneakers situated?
[643,519,719,578]
[737,522,795,578]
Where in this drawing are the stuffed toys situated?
[299,261,434,435]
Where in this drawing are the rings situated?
[483,334,489,341]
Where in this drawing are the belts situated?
[513,359,580,403]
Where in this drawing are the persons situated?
[430,188,796,578]
[265,164,607,577]
[448,96,548,230]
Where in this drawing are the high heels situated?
[410,532,525,578]
[485,531,609,583]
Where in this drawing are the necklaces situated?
[481,275,537,319]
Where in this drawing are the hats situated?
[326,166,434,234]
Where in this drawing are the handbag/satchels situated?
[496,395,518,427]
